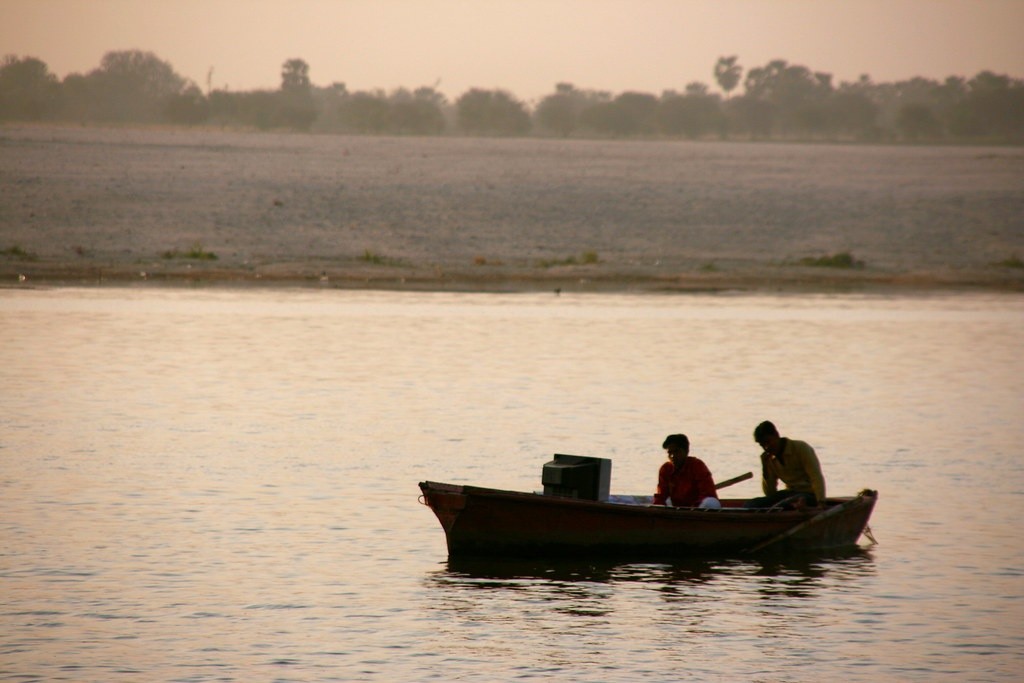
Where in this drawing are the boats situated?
[418,451,879,564]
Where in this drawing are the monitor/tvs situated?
[542,453,610,500]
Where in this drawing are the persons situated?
[753,421,825,512]
[653,434,718,508]
